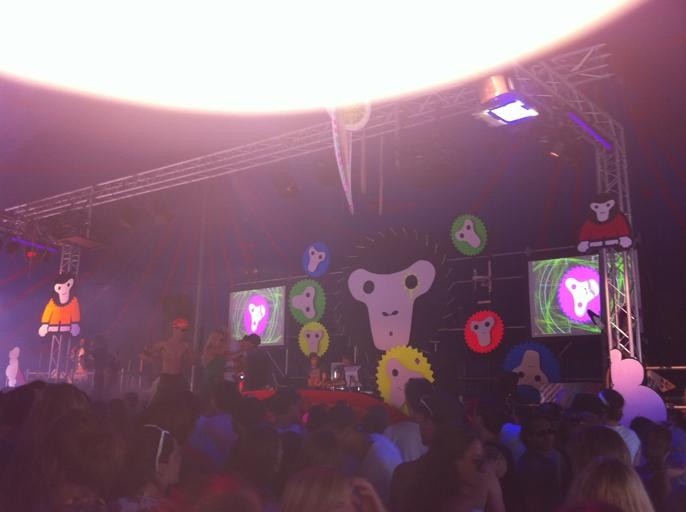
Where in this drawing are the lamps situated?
[471,69,541,126]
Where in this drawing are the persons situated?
[1,320,685,512]
